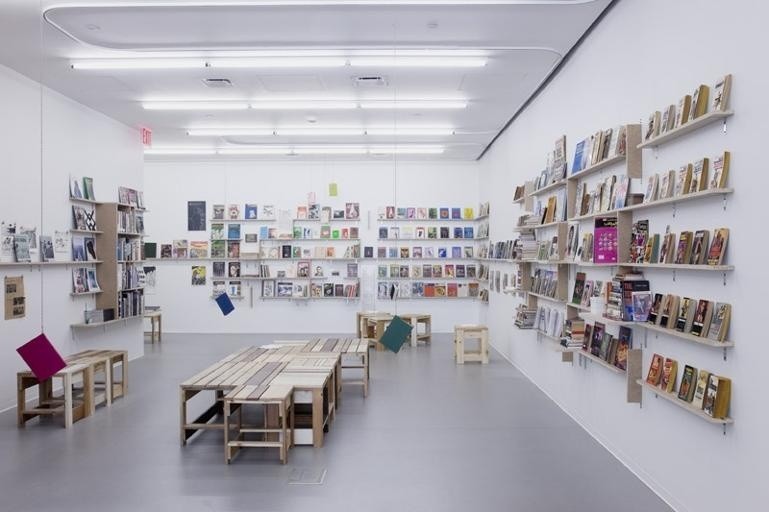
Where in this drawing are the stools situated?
[456,323,489,365]
[180,339,369,465]
[357,313,433,352]
[17,350,128,428]
[145,312,160,344]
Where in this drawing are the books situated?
[620,75,733,420]
[71,172,147,326]
[493,240,520,296]
[208,196,493,303]
[161,243,172,259]
[143,242,157,259]
[512,125,631,371]
[3,222,72,262]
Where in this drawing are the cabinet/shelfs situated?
[568,155,636,377]
[70,197,144,327]
[636,111,735,426]
[143,206,510,300]
[513,179,567,355]
